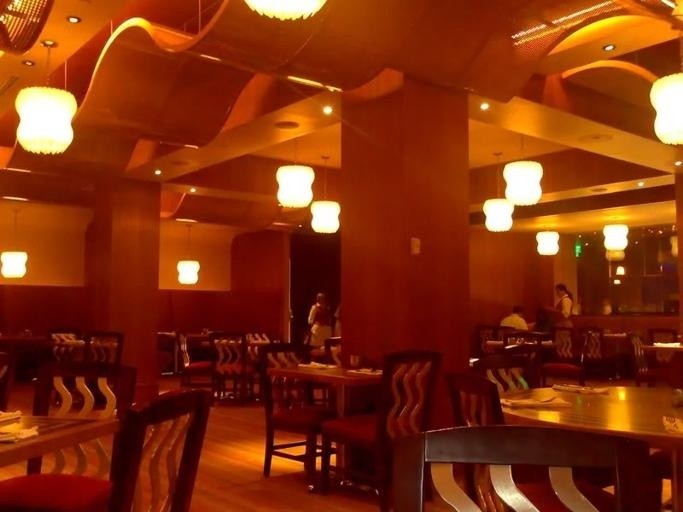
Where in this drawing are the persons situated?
[499,306,530,329]
[553,283,572,325]
[532,307,553,333]
[307,292,331,348]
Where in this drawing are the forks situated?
[506,395,557,404]
[665,414,680,430]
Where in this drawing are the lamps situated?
[602,216,629,250]
[0,210,26,278]
[647,72,682,146]
[15,40,74,154]
[481,151,514,234]
[174,225,199,285]
[501,131,544,206]
[534,228,559,257]
[309,155,339,234]
[275,137,315,208]
